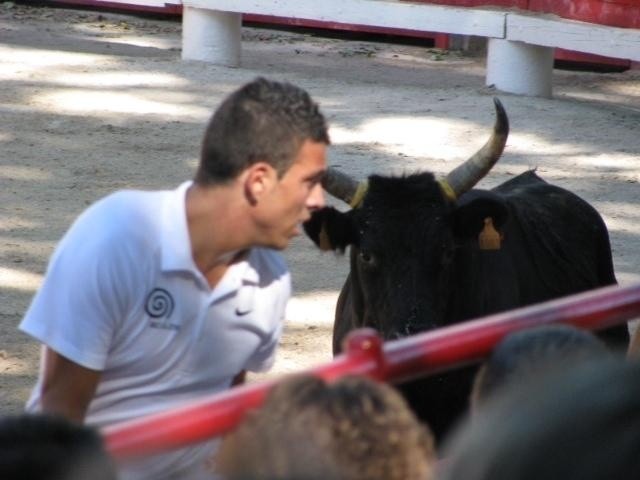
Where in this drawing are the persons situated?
[18,76,330,480]
[2,325,639,480]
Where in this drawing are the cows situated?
[303,96,633,445]
